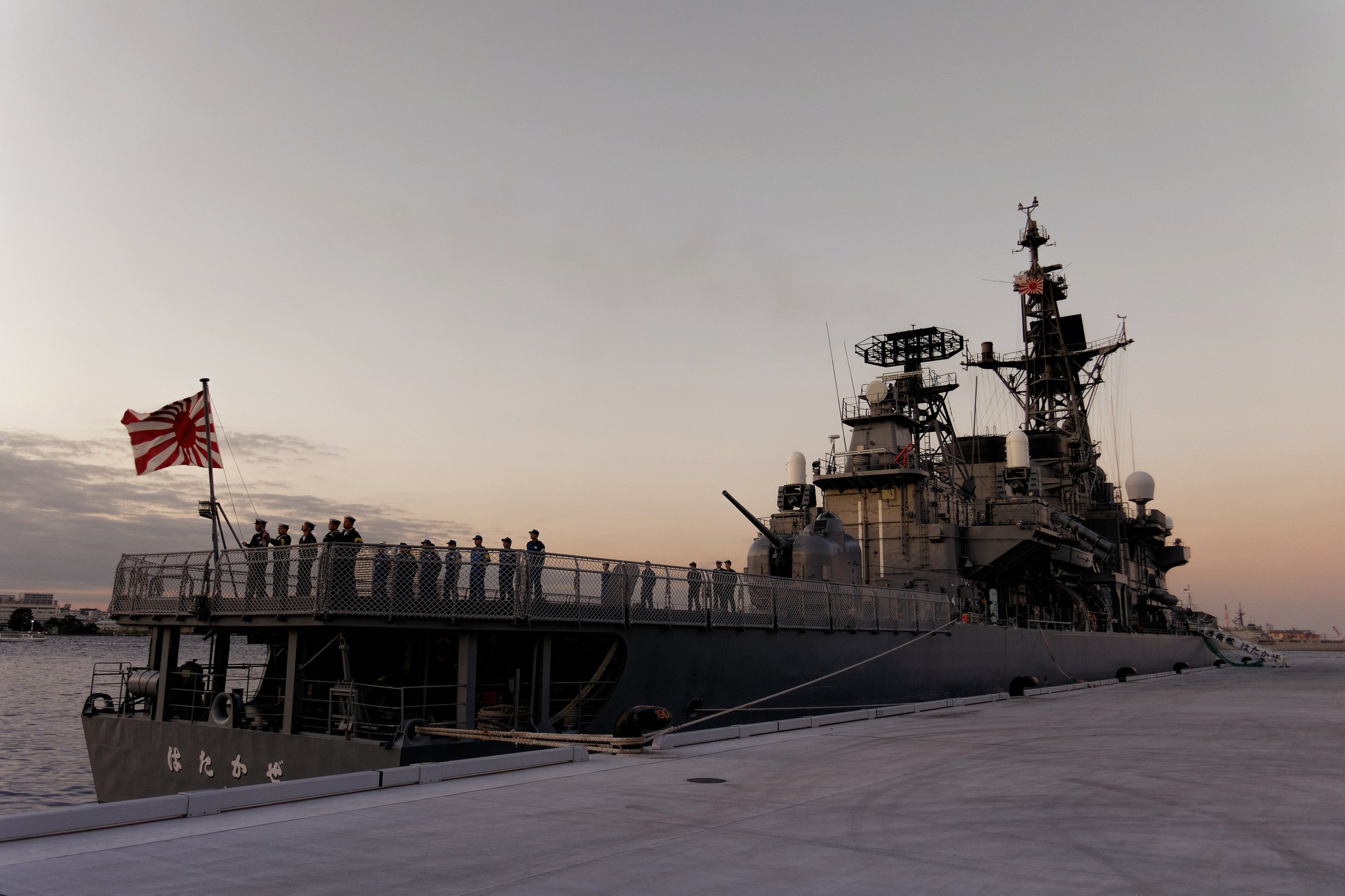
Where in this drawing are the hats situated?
[253,515,356,530]
[645,561,650,563]
[724,560,732,565]
[715,560,723,564]
[602,563,610,567]
[377,538,456,548]
[472,535,483,540]
[689,562,696,566]
[501,537,512,543]
[529,529,539,535]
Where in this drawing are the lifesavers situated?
[1091,614,1097,628]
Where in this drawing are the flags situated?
[121,389,224,476]
[1019,279,1043,295]
[1013,274,1026,283]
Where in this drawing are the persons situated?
[262,523,292,597]
[372,529,546,601]
[322,515,364,595]
[242,518,270,598]
[686,559,738,613]
[601,560,656,610]
[295,520,319,595]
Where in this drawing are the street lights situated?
[31,620,34,632]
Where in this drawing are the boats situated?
[0,633,47,642]
[1217,602,1345,651]
[80,196,1220,815]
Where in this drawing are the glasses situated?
[690,565,694,566]
[502,541,507,543]
[529,533,536,535]
